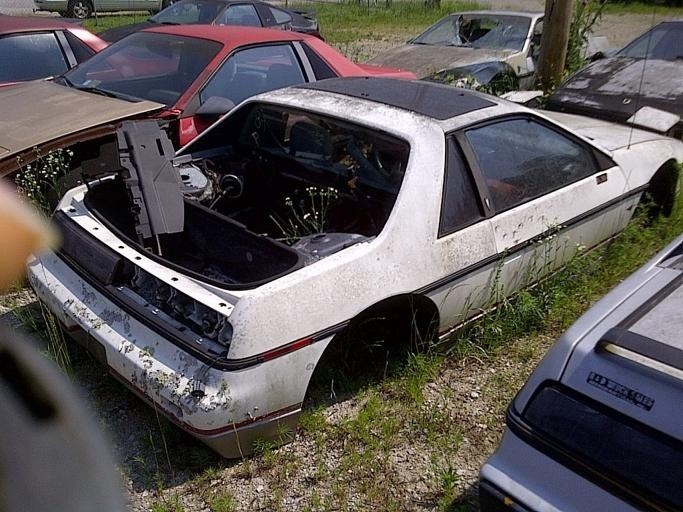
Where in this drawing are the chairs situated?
[197,5,218,24]
[288,120,328,152]
[171,54,298,100]
[241,13,261,26]
